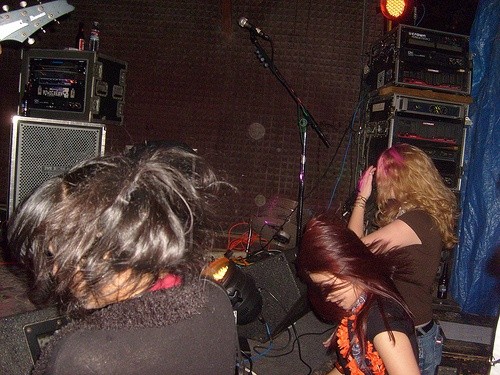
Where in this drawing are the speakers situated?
[0,304,70,375]
[236,252,310,343]
[7,114,106,221]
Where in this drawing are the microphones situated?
[238,16,272,40]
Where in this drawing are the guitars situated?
[0,0,75,45]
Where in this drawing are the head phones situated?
[259,223,290,251]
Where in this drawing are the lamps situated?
[380,0,418,20]
[202,259,262,326]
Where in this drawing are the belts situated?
[416,319,434,335]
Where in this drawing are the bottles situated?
[89,21,101,51]
[437,263,449,299]
[76,22,85,50]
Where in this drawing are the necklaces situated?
[338,304,385,375]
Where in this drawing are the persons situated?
[299,212,421,375]
[348,142,462,375]
[5,145,241,375]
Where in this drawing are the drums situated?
[251,196,315,248]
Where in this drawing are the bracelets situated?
[354,195,367,209]
